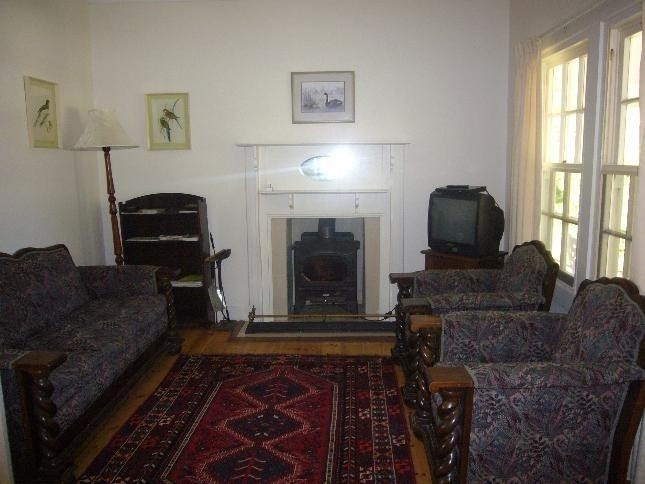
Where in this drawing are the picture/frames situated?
[22,74,61,149]
[142,90,191,153]
[287,68,356,125]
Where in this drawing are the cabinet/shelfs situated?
[419,249,508,270]
[117,192,216,329]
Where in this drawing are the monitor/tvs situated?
[428,190,504,256]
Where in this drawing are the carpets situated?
[67,351,418,484]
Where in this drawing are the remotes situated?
[447,185,469,188]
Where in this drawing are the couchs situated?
[1,242,181,483]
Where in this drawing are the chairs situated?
[412,277,644,484]
[385,238,560,400]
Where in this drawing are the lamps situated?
[71,107,141,265]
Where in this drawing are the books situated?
[178,209,199,213]
[160,233,199,242]
[173,273,203,288]
